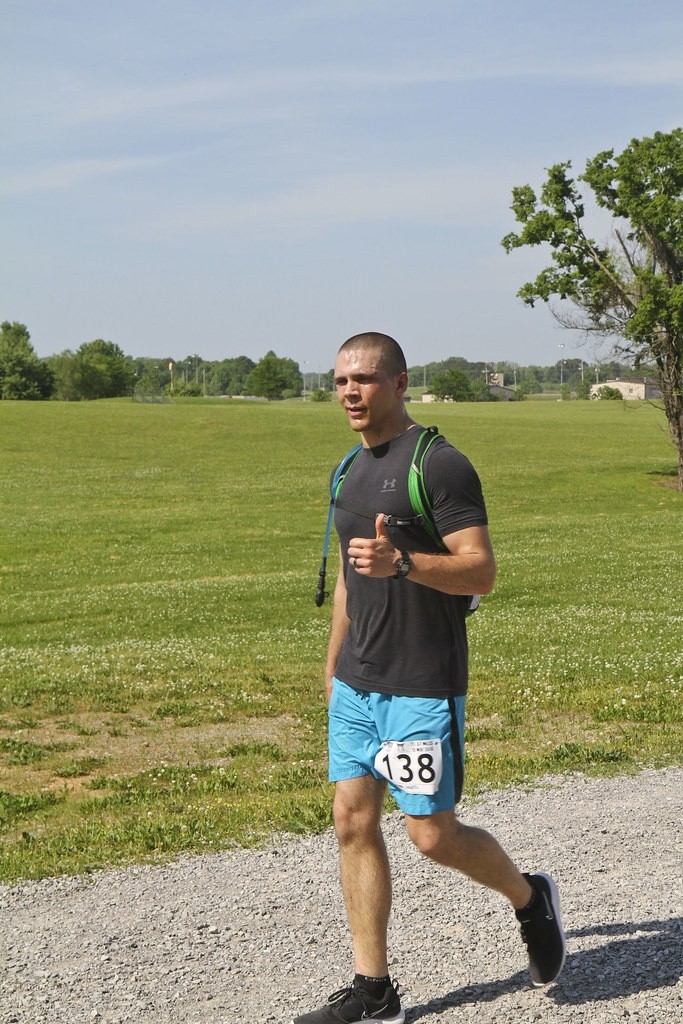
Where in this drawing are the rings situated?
[354,558,358,568]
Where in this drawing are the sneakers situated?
[291,977,406,1024]
[515,872,566,987]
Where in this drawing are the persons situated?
[291,332,565,1024]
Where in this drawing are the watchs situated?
[393,550,412,579]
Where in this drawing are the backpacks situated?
[315,425,481,620]
